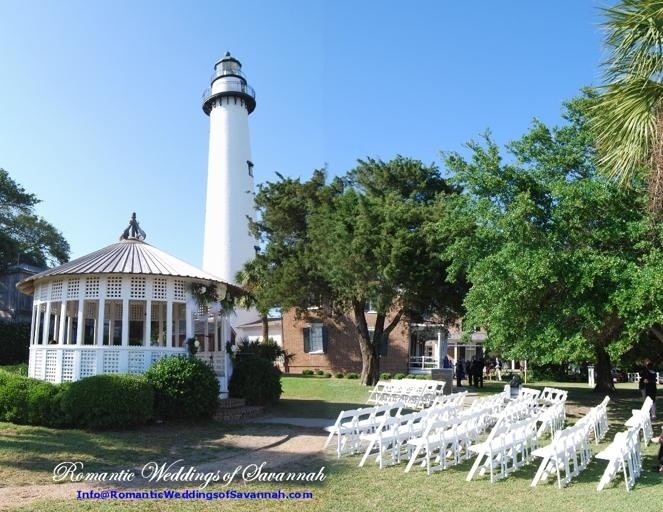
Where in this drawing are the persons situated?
[639,358,657,418]
[651,432,663,472]
[443,355,503,389]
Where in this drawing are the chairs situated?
[320,372,654,494]
